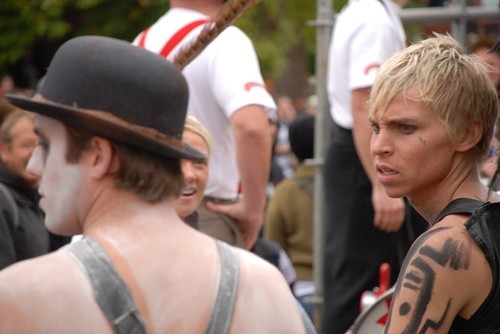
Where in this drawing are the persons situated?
[177,118,245,249]
[0,77,71,269]
[370,39,500,334]
[472,49,500,194]
[322,0,429,334]
[266,114,315,324]
[0,36,318,334]
[130,0,278,249]
[270,95,317,187]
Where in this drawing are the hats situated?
[5,36,207,159]
[290,117,314,158]
